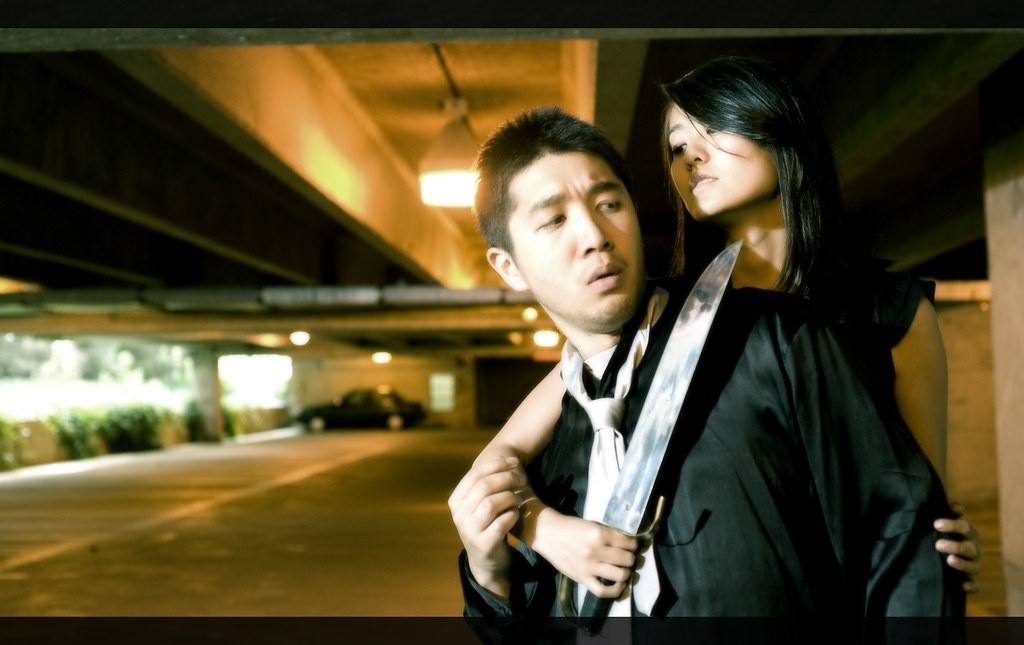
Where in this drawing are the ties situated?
[559,286,674,616]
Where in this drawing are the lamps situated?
[419,96,483,209]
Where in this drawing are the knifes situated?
[578,241,744,629]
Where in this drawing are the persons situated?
[447,52,983,645]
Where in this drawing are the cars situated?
[294,385,427,434]
